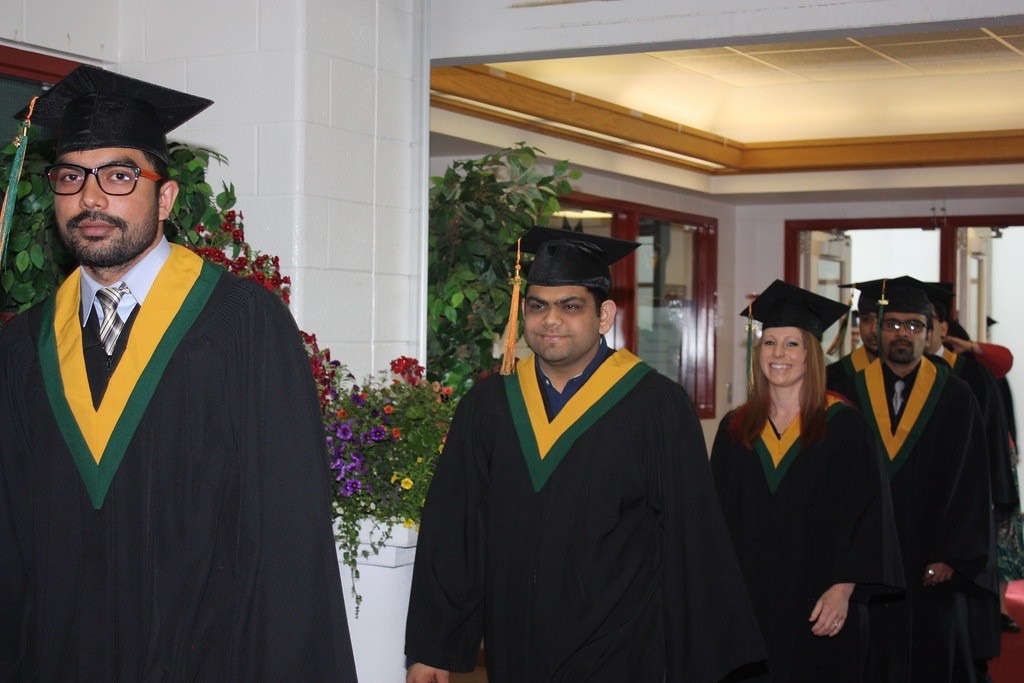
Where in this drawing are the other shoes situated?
[999,612,1020,633]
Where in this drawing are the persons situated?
[912,283,1020,547]
[826,280,894,408]
[0,62,358,683]
[987,316,1017,456]
[942,319,1024,633]
[403,225,771,683]
[838,278,1000,683]
[710,278,906,683]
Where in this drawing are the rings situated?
[928,568,935,576]
[833,622,838,628]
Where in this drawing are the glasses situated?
[879,317,929,334]
[43,160,164,197]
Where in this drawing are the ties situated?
[96,282,132,357]
[891,380,906,415]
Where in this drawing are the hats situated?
[497,224,643,377]
[0,63,218,278]
[738,278,853,403]
[826,275,956,359]
